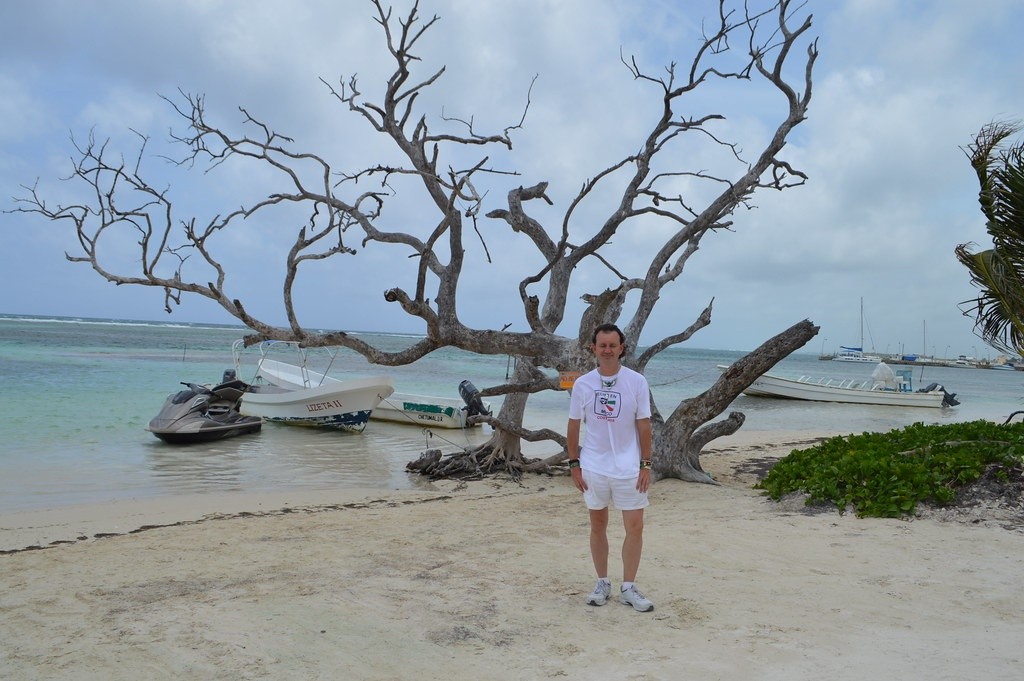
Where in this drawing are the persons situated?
[567,324,655,612]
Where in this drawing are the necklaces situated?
[598,365,619,390]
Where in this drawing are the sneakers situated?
[586,580,612,606]
[619,584,653,612]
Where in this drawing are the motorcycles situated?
[143,369,263,445]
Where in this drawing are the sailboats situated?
[830,296,881,364]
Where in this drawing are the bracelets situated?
[640,460,651,470]
[569,459,579,468]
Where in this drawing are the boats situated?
[716,357,962,409]
[256,339,492,430]
[199,367,395,435]
